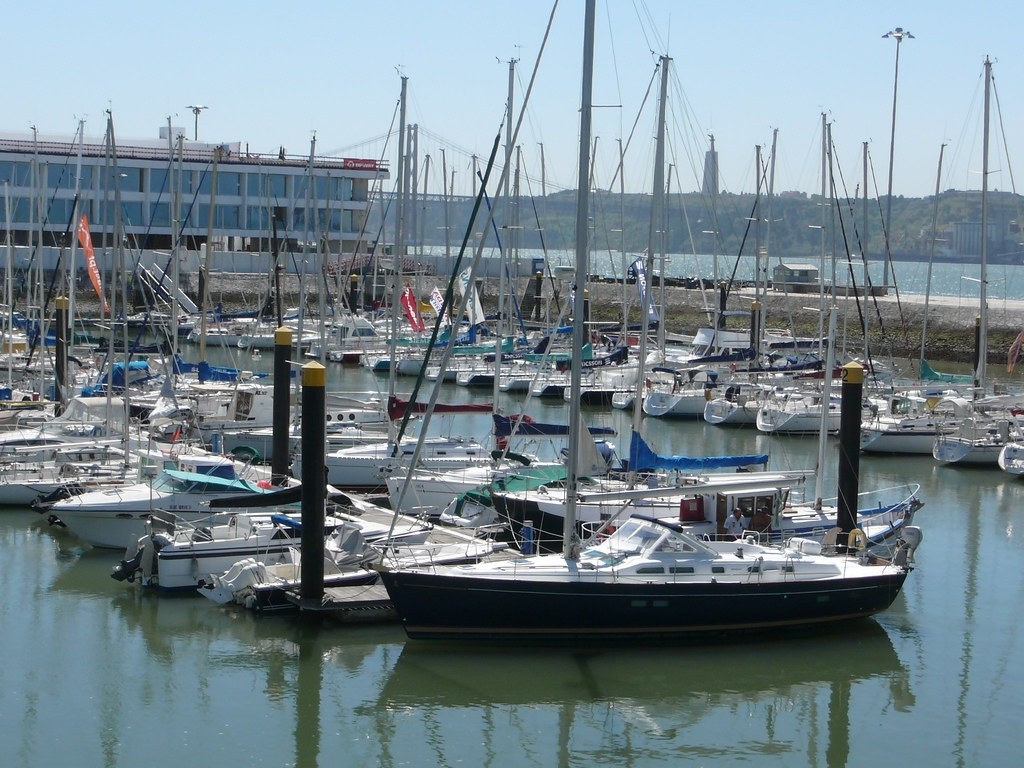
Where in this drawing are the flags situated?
[400,257,660,332]
[1007,331,1024,372]
[77,213,111,313]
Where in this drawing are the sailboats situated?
[1,0,1024,641]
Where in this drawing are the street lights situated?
[878,27,914,294]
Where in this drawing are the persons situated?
[748,506,774,541]
[723,508,748,542]
